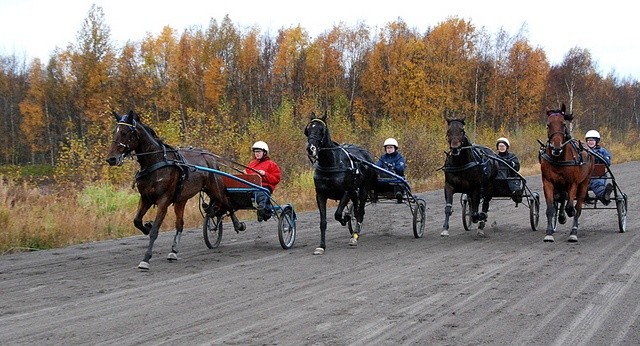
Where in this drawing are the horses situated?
[440,115,500,236]
[539,102,595,241]
[306,110,378,254]
[106,107,246,269]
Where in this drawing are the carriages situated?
[107,109,297,269]
[537,103,628,242]
[304,111,426,255]
[438,113,540,237]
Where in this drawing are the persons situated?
[483,137,523,203]
[581,129,612,203]
[202,140,280,222]
[371,138,407,203]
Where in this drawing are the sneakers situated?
[603,183,612,203]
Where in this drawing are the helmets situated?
[585,130,600,143]
[251,140,269,156]
[496,138,510,148]
[384,138,398,149]
